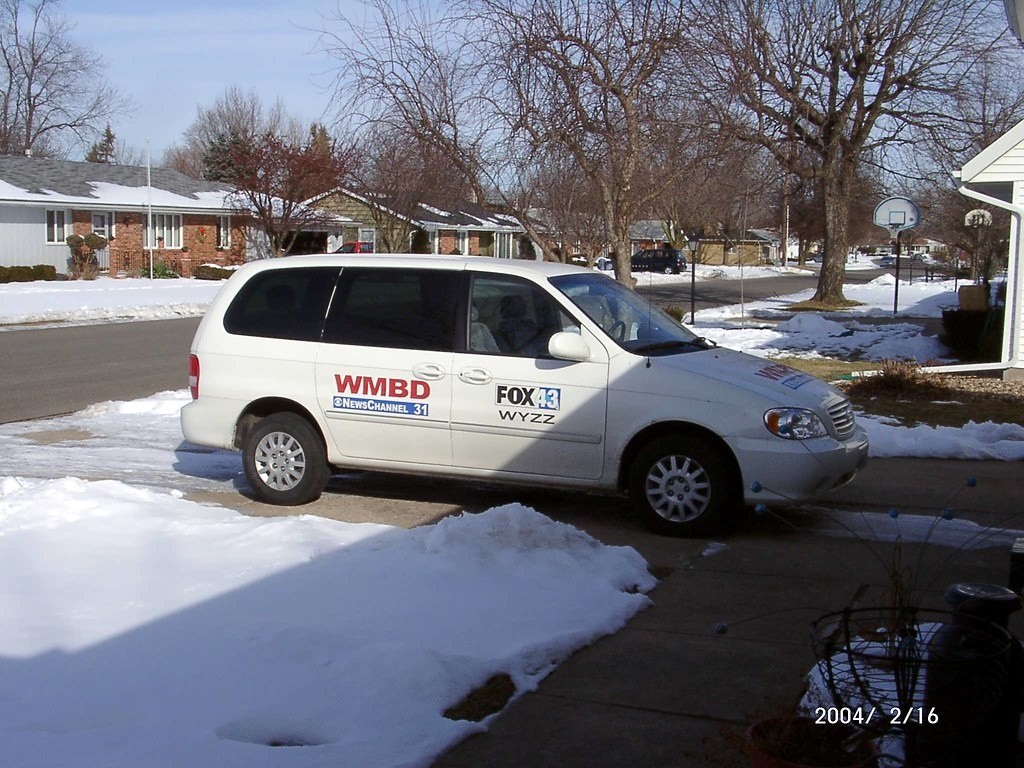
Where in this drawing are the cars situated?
[809,252,823,262]
[879,250,888,256]
[879,257,896,267]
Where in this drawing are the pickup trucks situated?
[333,242,373,253]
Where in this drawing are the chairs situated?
[493,294,547,358]
[470,305,501,354]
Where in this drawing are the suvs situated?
[631,248,687,274]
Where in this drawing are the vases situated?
[742,716,879,768]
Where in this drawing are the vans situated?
[179,251,869,539]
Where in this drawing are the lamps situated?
[124,216,134,227]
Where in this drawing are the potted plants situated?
[215,246,223,258]
[155,236,164,248]
[181,246,189,259]
